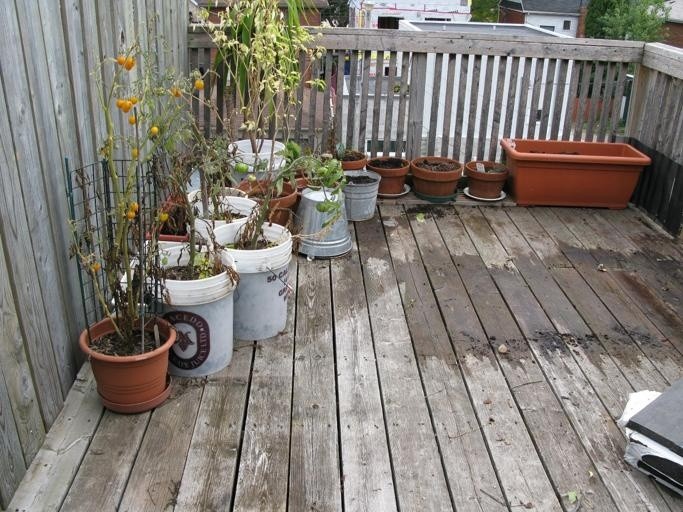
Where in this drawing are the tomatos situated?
[94,54,257,272]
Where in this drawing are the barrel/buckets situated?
[146,245,236,376]
[209,219,293,342]
[340,169,381,224]
[229,139,286,196]
[184,198,260,243]
[186,188,248,220]
[294,186,352,262]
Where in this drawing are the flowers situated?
[67,0,346,340]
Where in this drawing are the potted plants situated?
[334,169,383,222]
[80,142,351,416]
[320,146,367,172]
[408,156,464,199]
[499,140,651,210]
[367,155,411,197]
[462,160,511,203]
